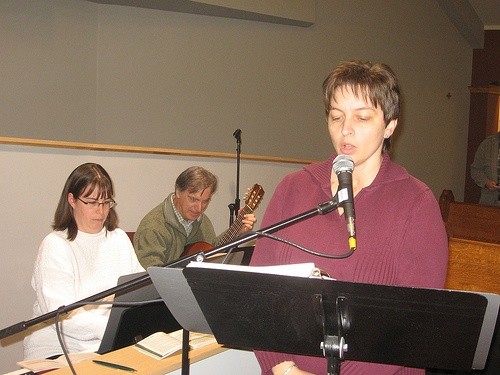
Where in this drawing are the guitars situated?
[179,183,265,258]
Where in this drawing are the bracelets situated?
[283,364,295,375]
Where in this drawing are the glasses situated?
[77,196,117,209]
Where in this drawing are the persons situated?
[133,166,257,272]
[22,163,147,360]
[248,59,449,375]
[471,131,500,207]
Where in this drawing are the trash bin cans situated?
[439,190,454,221]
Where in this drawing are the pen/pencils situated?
[93,360,137,373]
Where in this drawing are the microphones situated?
[333,154,357,252]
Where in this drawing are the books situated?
[133,328,217,360]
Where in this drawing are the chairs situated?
[437,187,500,298]
